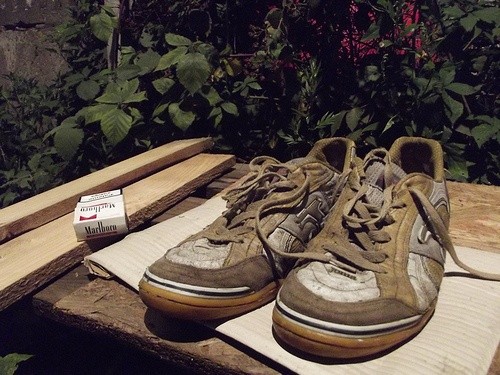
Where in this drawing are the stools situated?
[20,164,500,375]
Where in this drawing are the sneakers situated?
[138,138,358,321]
[271,135,500,359]
